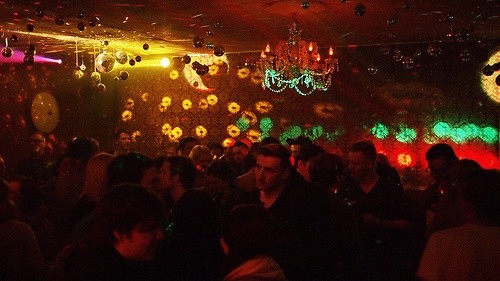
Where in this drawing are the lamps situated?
[253,24,339,95]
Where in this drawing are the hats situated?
[286,135,312,147]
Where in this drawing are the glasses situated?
[27,138,46,144]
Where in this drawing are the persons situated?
[0,129,426,281]
[428,160,483,237]
[416,170,500,281]
[422,144,459,225]
